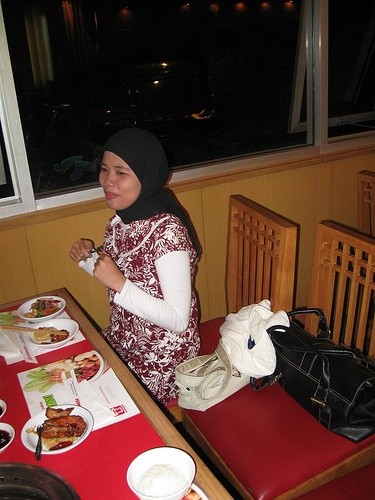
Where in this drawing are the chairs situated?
[166,171,375,500]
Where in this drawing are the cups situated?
[128,447,209,500]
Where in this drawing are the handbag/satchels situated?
[247,308,375,444]
[175,337,250,413]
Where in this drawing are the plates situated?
[0,399,7,420]
[27,318,79,349]
[21,404,94,455]
[18,296,67,323]
[0,422,15,454]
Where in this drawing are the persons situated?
[69,128,204,407]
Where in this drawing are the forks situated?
[35,423,44,461]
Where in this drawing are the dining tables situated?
[0,287,235,500]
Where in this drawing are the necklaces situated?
[112,215,171,264]
